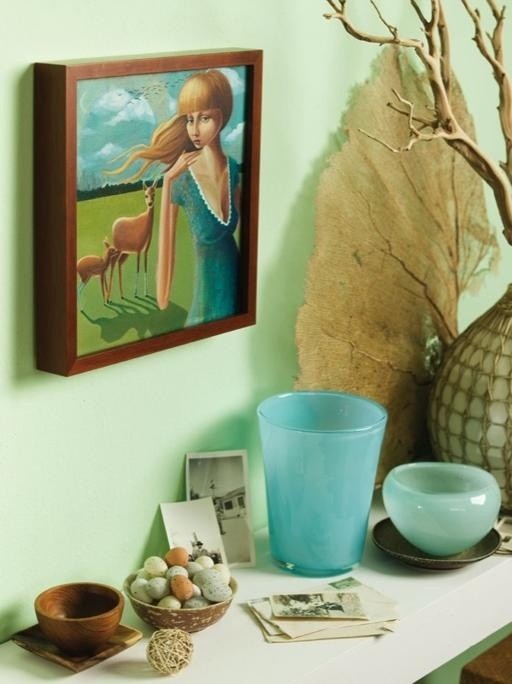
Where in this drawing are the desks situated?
[1,478,511,682]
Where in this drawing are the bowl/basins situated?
[381,459,505,555]
[33,581,125,652]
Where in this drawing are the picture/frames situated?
[28,41,264,376]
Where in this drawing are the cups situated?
[257,390,390,578]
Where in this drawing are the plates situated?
[368,519,502,576]
[15,616,143,674]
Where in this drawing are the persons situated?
[287,600,347,614]
[101,67,250,327]
[213,499,226,535]
[188,553,194,561]
[194,540,210,557]
[210,553,218,563]
